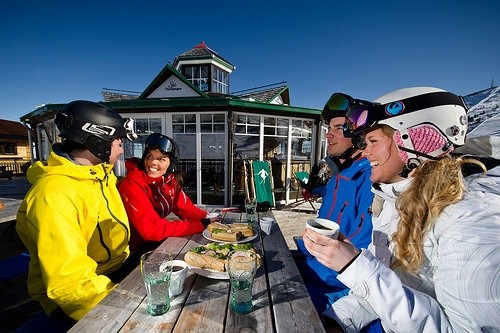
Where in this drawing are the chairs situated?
[292,171,322,211]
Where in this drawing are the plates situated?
[203,224,259,244]
[184,245,260,280]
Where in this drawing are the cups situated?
[226,249,257,314]
[260,217,274,236]
[306,218,340,246]
[206,212,220,218]
[140,249,173,316]
[245,198,257,223]
[159,260,187,296]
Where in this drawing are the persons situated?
[119,133,222,273]
[290,92,375,310]
[16,100,138,333]
[319,86,500,333]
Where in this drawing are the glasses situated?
[321,92,354,122]
[342,99,378,138]
[123,117,138,142]
[146,136,173,154]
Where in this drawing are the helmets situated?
[367,87,468,148]
[144,132,180,170]
[55,100,128,161]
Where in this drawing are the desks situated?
[65,210,325,333]
